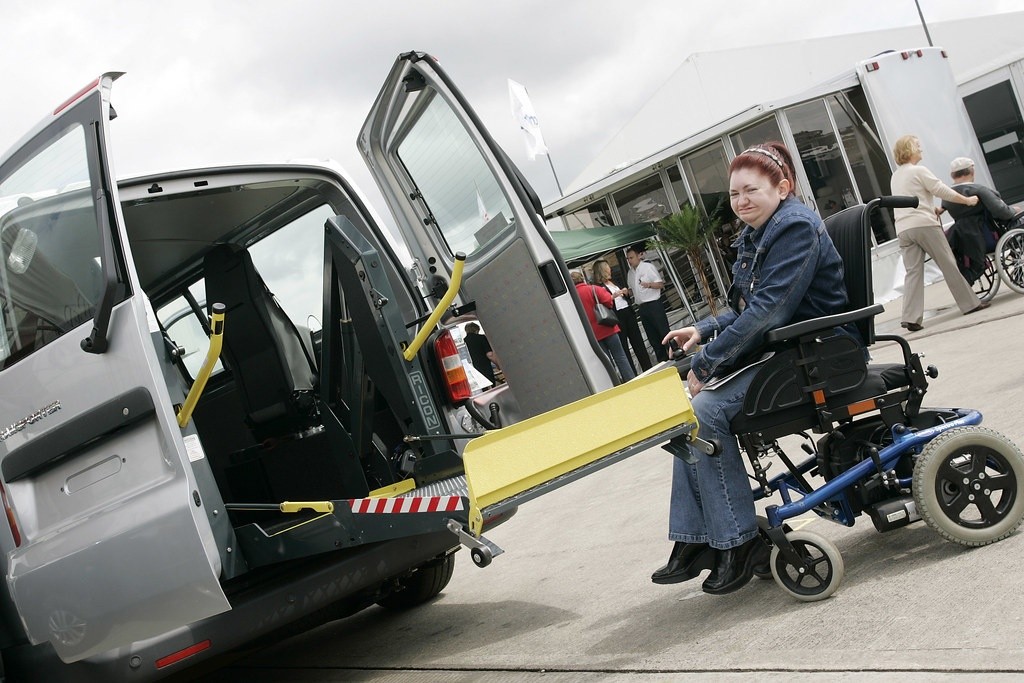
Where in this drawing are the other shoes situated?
[900,321,924,332]
[964,303,991,315]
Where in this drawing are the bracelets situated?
[649,282,652,288]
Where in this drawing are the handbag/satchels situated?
[591,284,620,328]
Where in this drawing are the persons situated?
[890,135,991,332]
[650,141,868,595]
[626,246,672,364]
[941,156,1024,253]
[592,259,653,378]
[571,271,635,382]
[463,322,501,392]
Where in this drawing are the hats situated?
[950,158,975,171]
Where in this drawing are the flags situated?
[508,78,549,162]
[475,190,490,229]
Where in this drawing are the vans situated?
[1,45,702,683]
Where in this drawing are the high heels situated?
[651,541,717,584]
[702,533,772,595]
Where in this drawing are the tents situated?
[549,220,697,325]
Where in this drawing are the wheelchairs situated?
[651,194,1023,602]
[936,195,1024,306]
